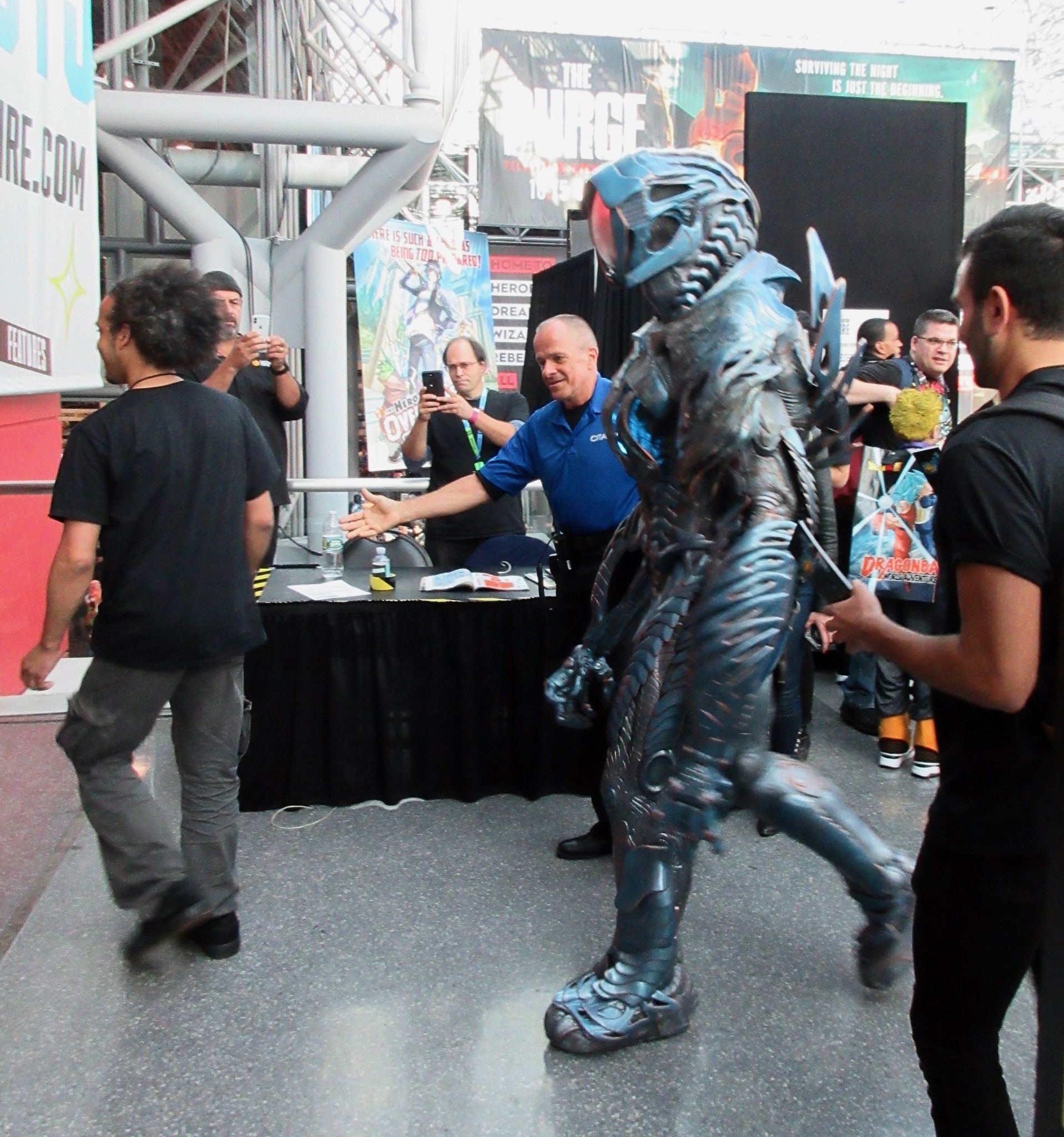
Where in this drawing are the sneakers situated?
[840,705,879,736]
[180,911,241,959]
[127,880,214,963]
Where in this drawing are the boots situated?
[911,719,941,778]
[878,712,916,768]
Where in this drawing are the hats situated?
[199,271,242,297]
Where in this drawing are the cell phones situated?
[802,623,825,650]
[249,313,271,353]
[784,519,852,602]
[421,370,447,413]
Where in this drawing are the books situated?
[418,567,557,593]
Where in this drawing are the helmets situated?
[588,149,762,290]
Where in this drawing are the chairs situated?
[463,534,556,566]
[342,530,433,567]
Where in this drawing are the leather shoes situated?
[557,831,612,860]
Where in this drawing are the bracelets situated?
[269,364,289,375]
[468,407,481,425]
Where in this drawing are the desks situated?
[236,565,595,814]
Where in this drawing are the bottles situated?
[373,547,391,577]
[323,510,345,577]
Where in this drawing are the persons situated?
[175,271,309,572]
[821,201,1063,1137]
[743,309,962,839]
[16,263,282,961]
[539,150,921,1057]
[401,337,530,570]
[342,315,668,860]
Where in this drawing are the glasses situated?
[444,361,481,372]
[917,336,959,351]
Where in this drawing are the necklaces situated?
[129,372,179,390]
